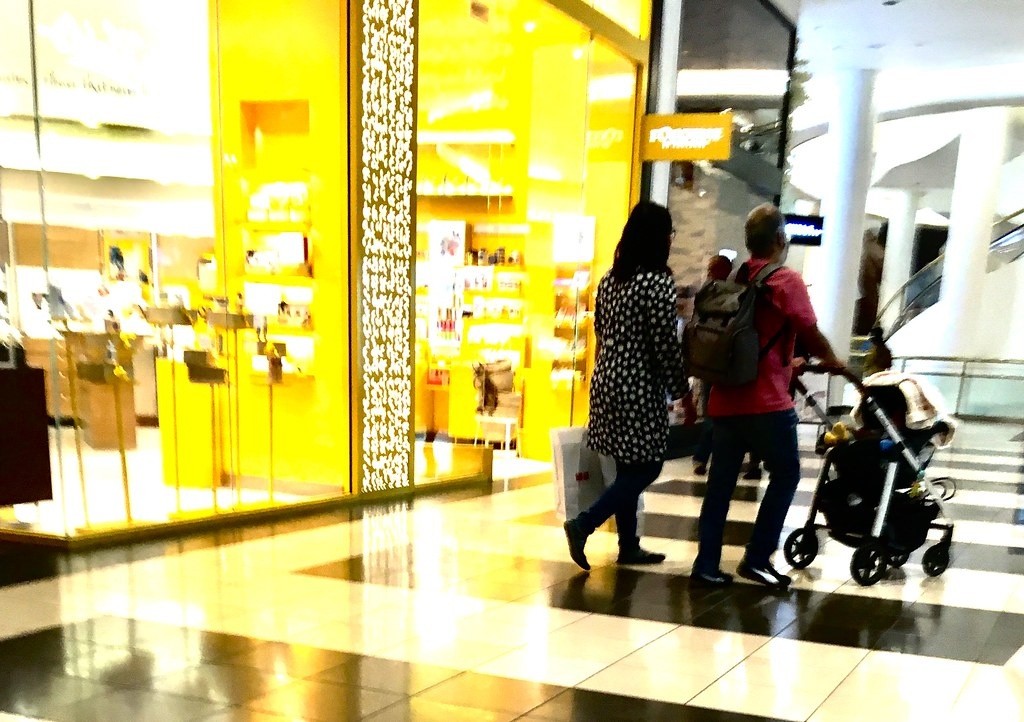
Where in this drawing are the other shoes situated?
[689,568,733,586]
[564,520,590,570]
[618,549,665,563]
[736,551,791,587]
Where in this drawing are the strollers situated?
[783,362,957,585]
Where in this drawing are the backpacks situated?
[682,261,794,389]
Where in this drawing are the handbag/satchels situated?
[550,427,645,521]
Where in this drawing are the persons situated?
[862,325,893,380]
[669,254,809,480]
[689,202,848,588]
[562,201,697,572]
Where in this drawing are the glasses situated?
[670,229,676,238]
